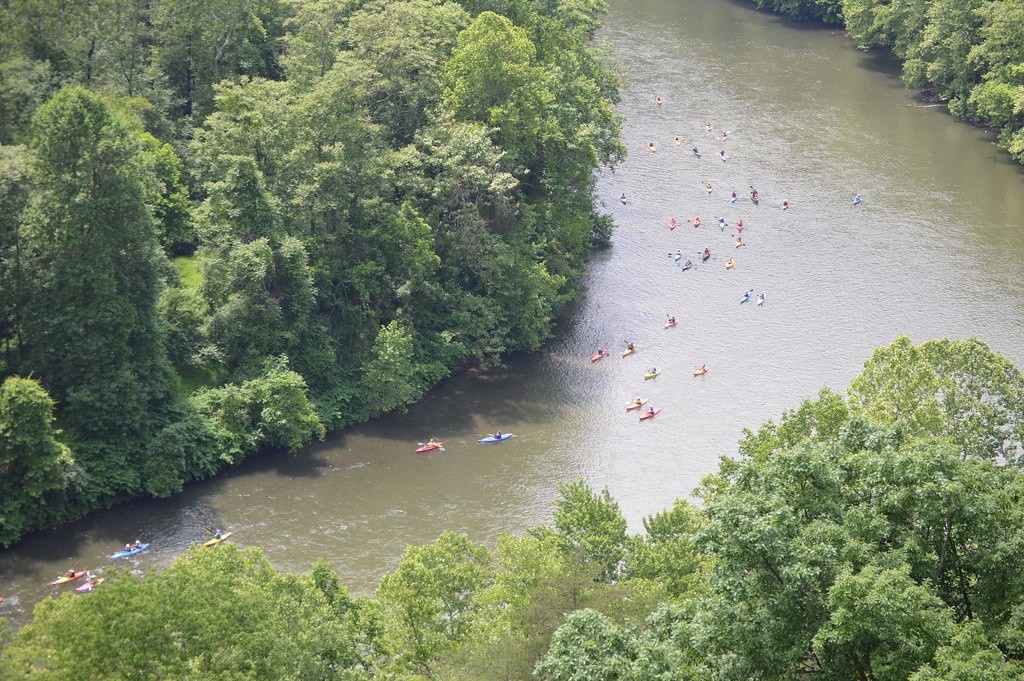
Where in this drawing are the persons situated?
[125,543,131,552]
[65,569,76,578]
[87,576,97,589]
[589,95,793,420]
[426,439,436,449]
[853,193,864,205]
[213,528,224,542]
[493,430,503,440]
[134,540,142,550]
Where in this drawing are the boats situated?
[643,368,662,380]
[621,343,638,359]
[621,199,627,204]
[674,253,682,261]
[625,398,649,411]
[640,407,661,421]
[702,250,711,260]
[592,347,609,362]
[682,262,693,273]
[111,542,150,560]
[725,259,735,270]
[203,532,232,547]
[75,576,106,592]
[756,293,766,305]
[48,568,89,585]
[477,432,515,445]
[646,96,789,249]
[740,290,754,304]
[663,320,679,330]
[854,196,861,204]
[414,441,444,452]
[694,367,709,377]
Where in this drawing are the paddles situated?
[750,186,761,199]
[714,215,728,226]
[698,252,714,255]
[731,234,745,246]
[418,443,443,449]
[668,253,681,255]
[624,340,629,344]
[666,313,676,328]
